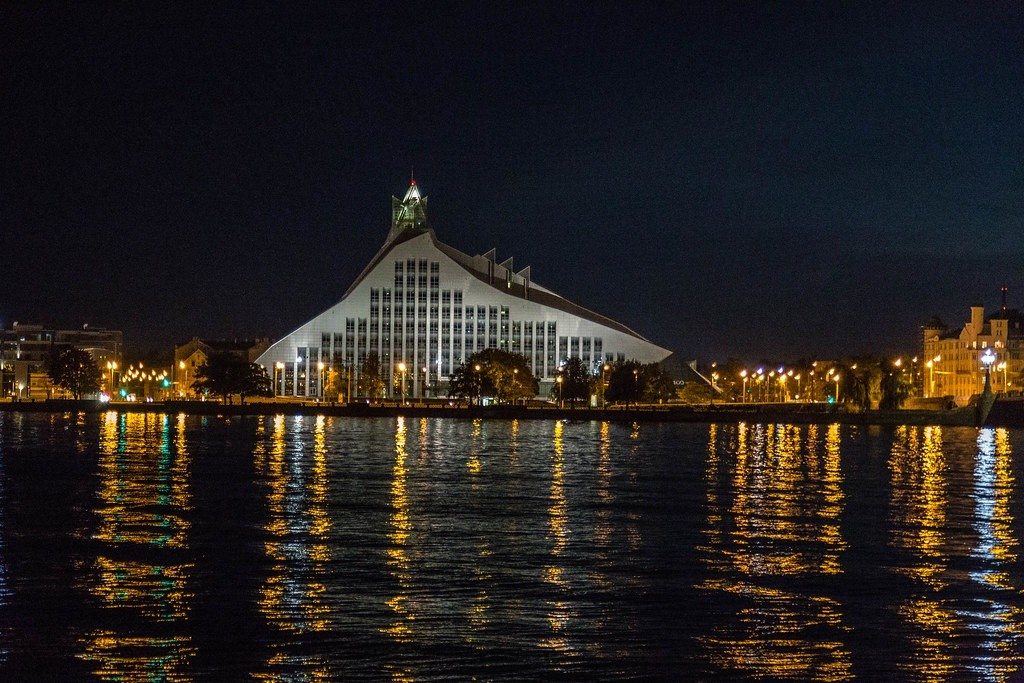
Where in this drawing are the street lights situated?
[982,349,995,394]
[603,365,610,407]
[275,362,284,398]
[318,363,325,402]
[741,366,774,403]
[558,366,563,408]
[513,369,518,405]
[399,363,405,404]
